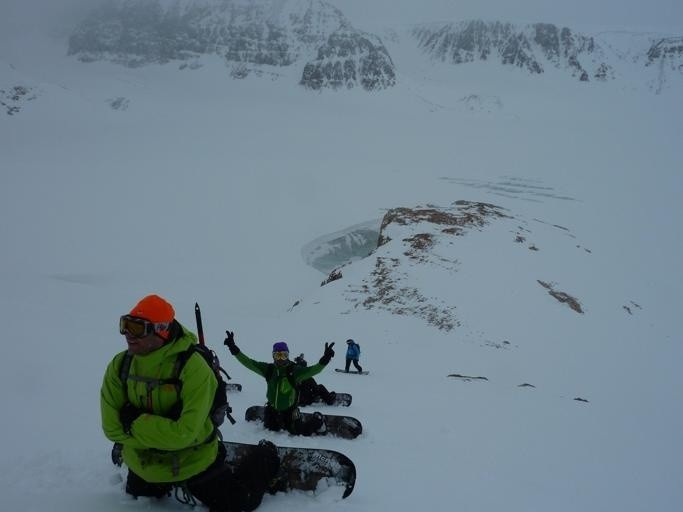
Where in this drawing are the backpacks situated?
[118,343,236,441]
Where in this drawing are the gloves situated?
[221,328,240,358]
[117,399,148,436]
[167,400,185,421]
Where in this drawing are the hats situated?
[271,340,289,351]
[127,294,176,338]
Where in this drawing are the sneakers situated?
[312,410,329,435]
[256,437,280,486]
[327,390,337,406]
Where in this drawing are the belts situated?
[318,340,335,366]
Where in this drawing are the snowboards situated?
[245,406,361,439]
[112,443,356,499]
[311,393,352,406]
[335,369,369,375]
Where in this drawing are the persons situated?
[222,329,336,437]
[290,356,335,407]
[98,293,280,511]
[344,339,362,374]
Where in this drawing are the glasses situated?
[270,350,289,361]
[116,313,168,338]
